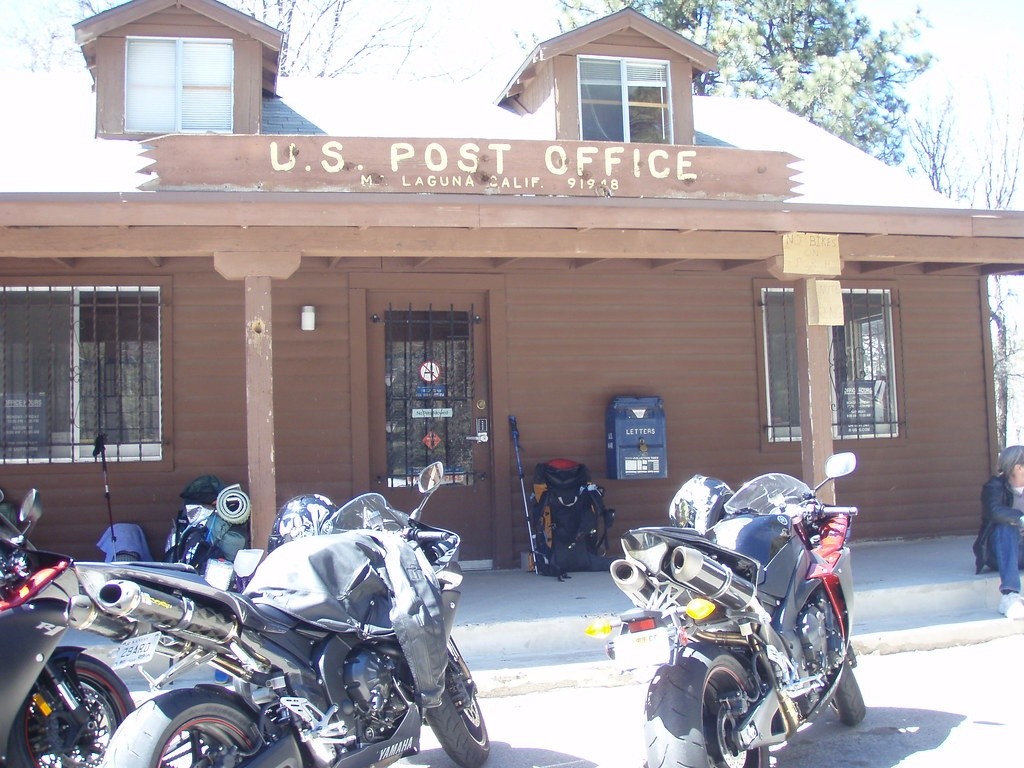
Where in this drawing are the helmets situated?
[669,473,733,536]
[267,492,338,555]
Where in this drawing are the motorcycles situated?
[64,459,492,768]
[588,451,867,768]
[0,487,137,768]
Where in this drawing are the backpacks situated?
[531,458,618,582]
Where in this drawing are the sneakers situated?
[998,592,1024,620]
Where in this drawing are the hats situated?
[996,445,1024,478]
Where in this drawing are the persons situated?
[972,445,1024,620]
